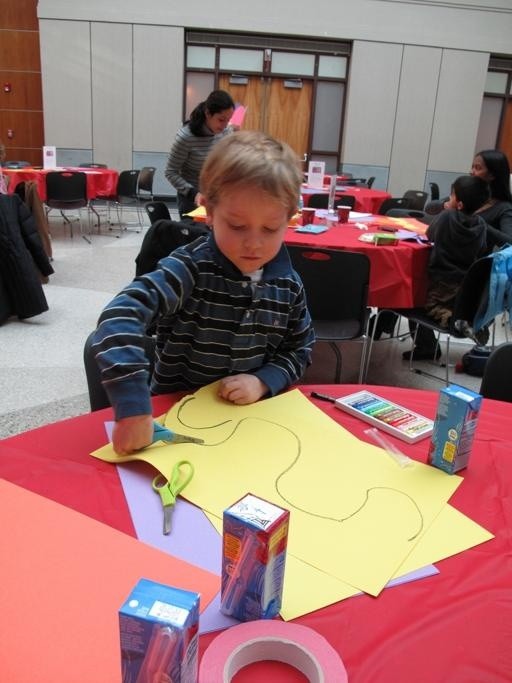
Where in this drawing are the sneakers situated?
[368,316,383,341]
[457,320,489,346]
[402,345,441,360]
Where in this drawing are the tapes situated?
[198,618,348,683]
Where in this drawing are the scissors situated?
[152,460,194,535]
[153,421,204,443]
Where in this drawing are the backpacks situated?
[461,346,496,378]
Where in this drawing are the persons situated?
[90,133,316,454]
[164,90,235,224]
[364,175,491,341]
[368,150,512,361]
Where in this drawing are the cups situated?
[302,210,314,225]
[338,206,351,223]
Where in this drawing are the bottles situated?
[299,196,303,208]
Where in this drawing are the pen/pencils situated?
[351,393,432,437]
[311,392,336,403]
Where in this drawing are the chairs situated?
[1,159,162,324]
